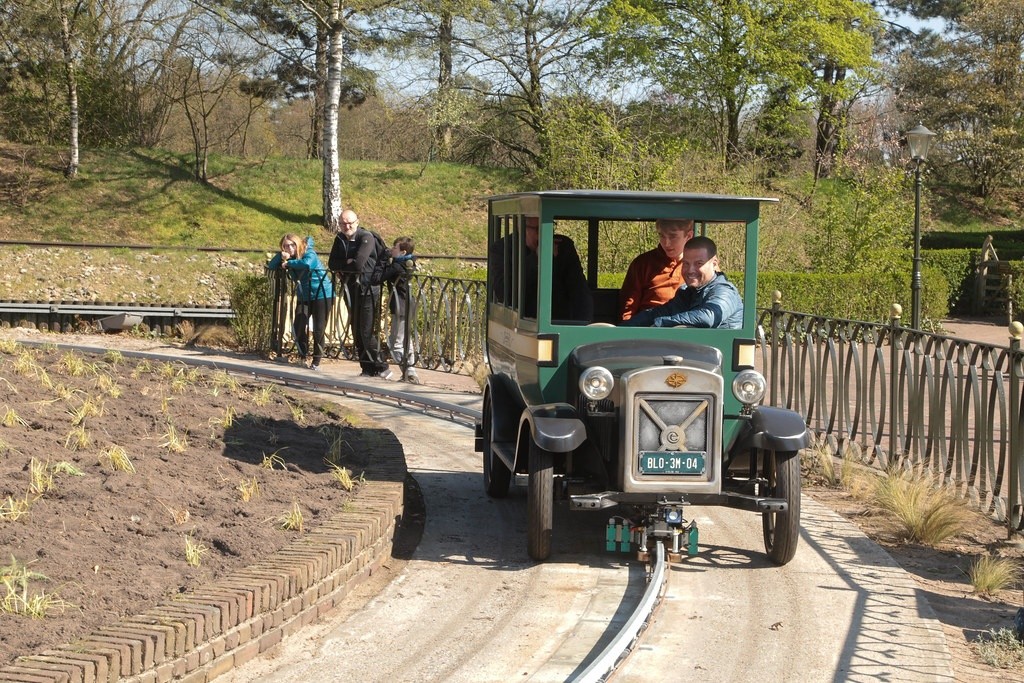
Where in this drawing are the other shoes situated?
[310,363,320,370]
[378,368,393,380]
[408,374,419,385]
[397,376,405,382]
[358,371,377,378]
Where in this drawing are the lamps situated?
[96,314,144,333]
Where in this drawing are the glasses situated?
[338,220,357,226]
[284,244,295,249]
[526,220,558,234]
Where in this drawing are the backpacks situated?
[355,227,390,287]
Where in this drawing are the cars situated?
[474,190,809,564]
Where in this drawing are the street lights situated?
[905,118,938,342]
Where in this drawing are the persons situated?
[371,236,419,384]
[881,129,907,166]
[493,218,591,326]
[268,233,336,370]
[328,209,390,376]
[618,217,744,329]
